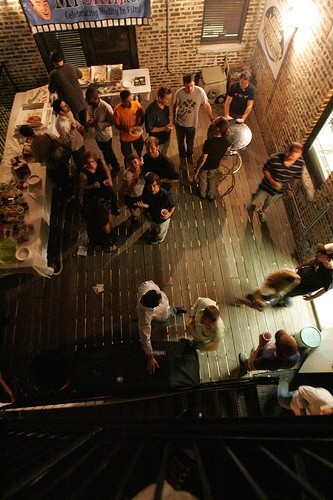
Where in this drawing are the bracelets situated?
[210,118,215,121]
[147,355,154,358]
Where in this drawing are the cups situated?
[79,125,84,134]
[167,123,173,129]
[263,331,271,341]
[146,171,153,176]
[87,117,94,126]
[160,208,168,217]
[103,180,110,186]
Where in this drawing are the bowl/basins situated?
[111,87,116,92]
[104,86,110,93]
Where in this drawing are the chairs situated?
[197,51,229,104]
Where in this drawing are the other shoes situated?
[150,238,161,245]
[175,307,188,315]
[239,351,249,369]
[257,206,266,223]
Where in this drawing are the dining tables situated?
[0,83,57,282]
[80,67,151,104]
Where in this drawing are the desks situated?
[206,117,253,196]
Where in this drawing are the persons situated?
[272,242,333,308]
[192,116,231,201]
[172,73,221,166]
[19,123,76,194]
[179,297,226,354]
[52,100,88,175]
[144,85,175,157]
[29,0,52,21]
[113,89,145,167]
[84,87,120,177]
[78,134,181,252]
[223,70,256,123]
[46,51,90,134]
[135,279,187,374]
[276,380,333,417]
[247,141,305,223]
[238,329,301,374]
[229,268,302,313]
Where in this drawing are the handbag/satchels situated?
[45,132,71,163]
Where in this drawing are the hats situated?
[275,329,297,357]
[317,243,333,259]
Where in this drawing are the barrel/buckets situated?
[293,326,321,348]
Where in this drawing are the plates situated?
[129,126,144,137]
[27,175,41,185]
[121,80,133,89]
[14,246,30,261]
